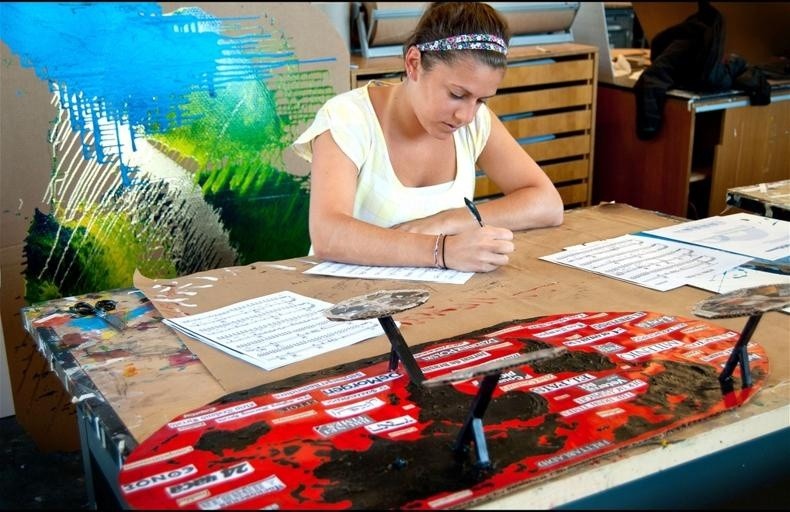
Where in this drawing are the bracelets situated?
[442,234,450,269]
[433,233,446,270]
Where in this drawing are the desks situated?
[592,65,790,218]
[21,203,790,510]
[726,179,790,222]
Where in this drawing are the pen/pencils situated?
[464,198,485,228]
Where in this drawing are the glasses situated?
[69,299,118,319]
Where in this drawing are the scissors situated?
[75,300,126,332]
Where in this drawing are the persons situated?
[279,1,566,274]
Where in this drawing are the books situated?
[160,290,402,373]
